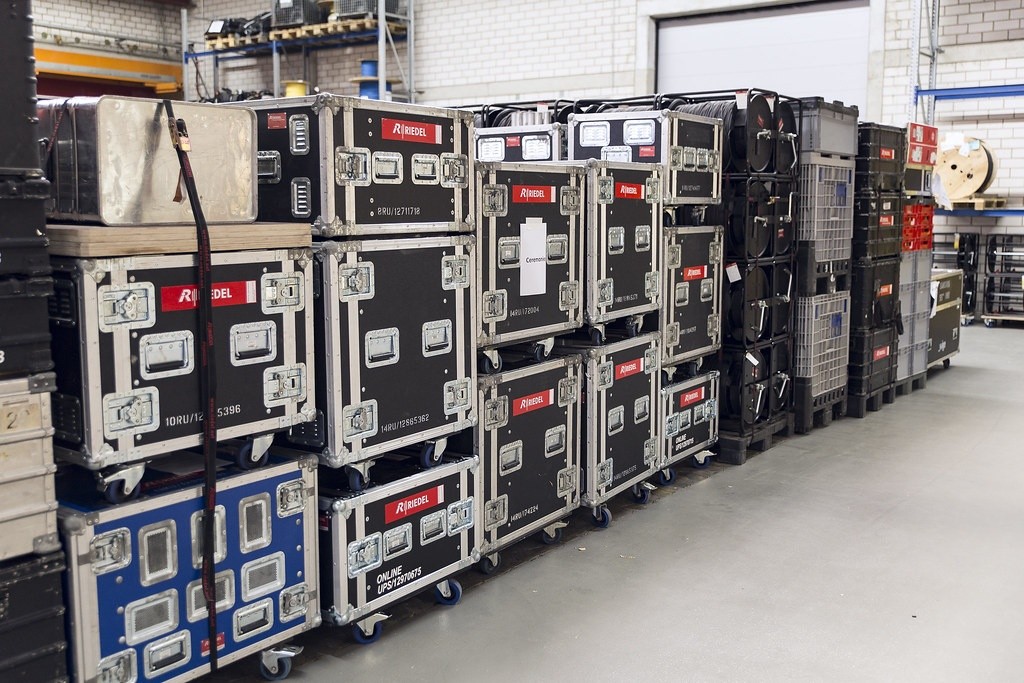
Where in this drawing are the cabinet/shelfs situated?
[210,0,414,103]
[909,0,1024,220]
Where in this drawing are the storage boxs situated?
[0,0,1024,683]
